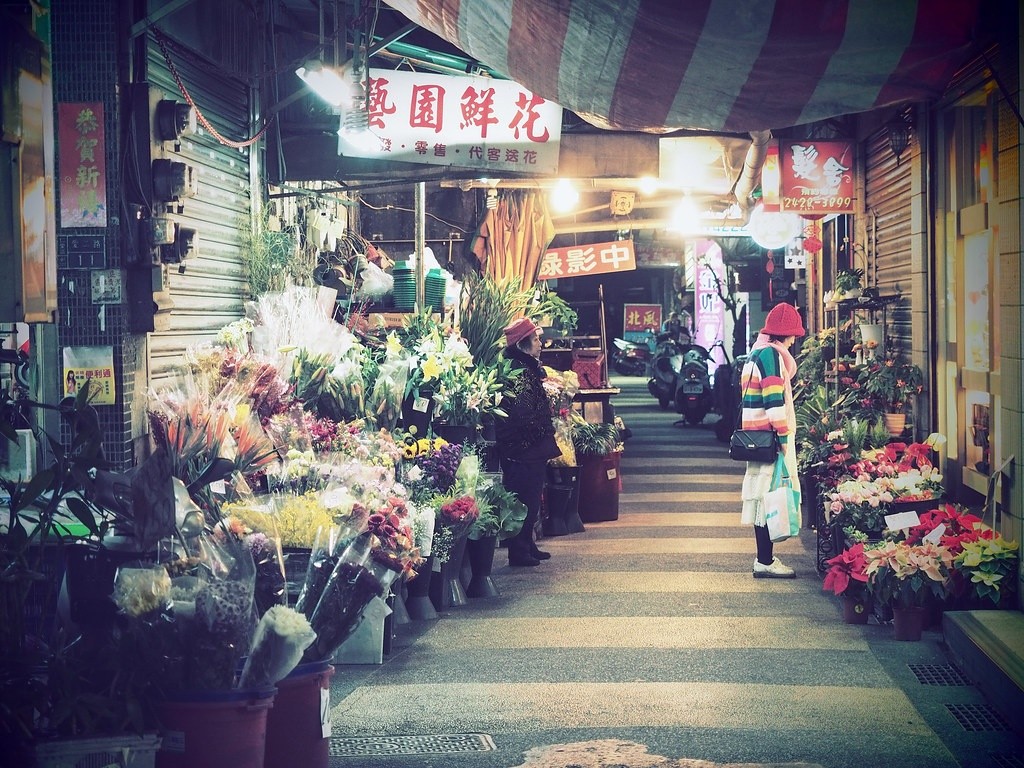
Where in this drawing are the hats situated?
[503,317,542,346]
[760,303,806,338]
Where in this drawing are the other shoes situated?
[509,553,541,567]
[528,544,552,560]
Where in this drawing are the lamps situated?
[296,60,353,106]
[883,108,915,165]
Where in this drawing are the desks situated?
[570,387,621,422]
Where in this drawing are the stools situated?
[569,348,610,390]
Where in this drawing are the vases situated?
[463,534,496,598]
[403,556,442,621]
[882,413,906,435]
[802,462,819,529]
[27,579,409,768]
[446,535,471,607]
[437,426,476,446]
[543,484,571,536]
[858,288,877,303]
[855,326,882,365]
[549,465,587,534]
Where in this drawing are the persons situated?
[741,302,806,579]
[492,318,563,567]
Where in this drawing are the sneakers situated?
[753,558,797,579]
[753,555,791,573]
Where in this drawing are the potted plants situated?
[572,421,621,523]
[831,269,868,302]
[820,504,1019,643]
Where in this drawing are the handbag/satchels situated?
[729,429,780,464]
[762,451,801,544]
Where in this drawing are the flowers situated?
[110,186,577,692]
[840,238,876,288]
[793,326,943,532]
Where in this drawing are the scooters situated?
[613,314,686,404]
[664,336,724,429]
[712,339,751,442]
